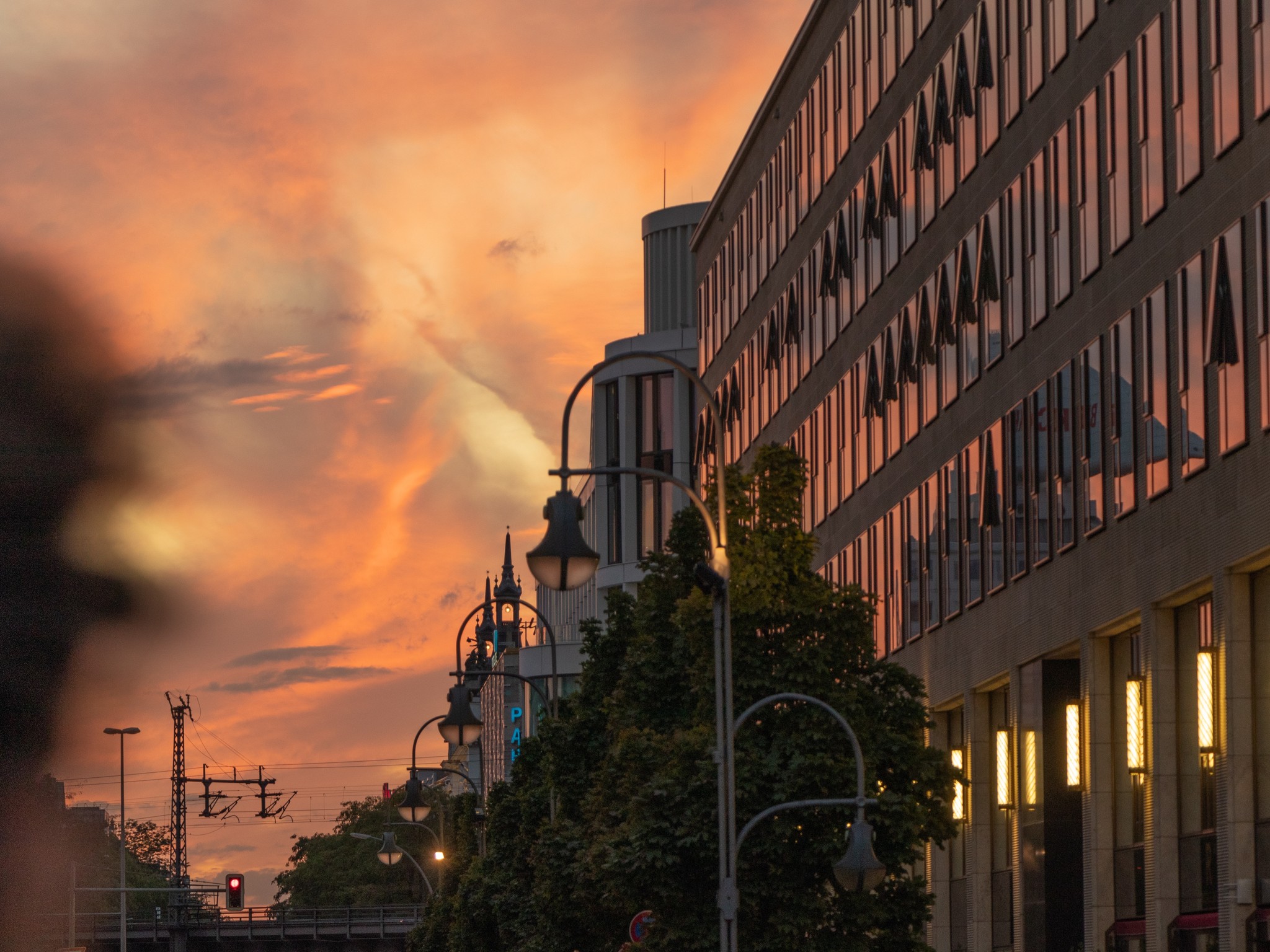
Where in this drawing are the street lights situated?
[102,727,141,952]
[393,715,489,859]
[378,780,445,898]
[435,597,561,825]
[521,350,891,952]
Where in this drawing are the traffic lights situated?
[225,873,245,911]
